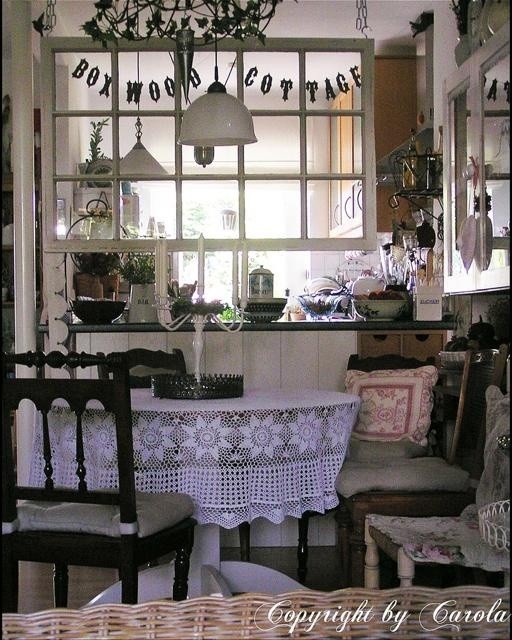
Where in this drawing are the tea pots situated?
[405,146,444,189]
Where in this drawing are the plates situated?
[307,277,340,294]
[86,159,113,188]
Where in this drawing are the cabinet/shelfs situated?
[389,149,442,209]
[442,18,512,300]
[328,67,363,238]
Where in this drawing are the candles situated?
[150,234,251,309]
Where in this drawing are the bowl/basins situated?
[351,299,408,321]
[295,294,345,320]
[69,300,126,325]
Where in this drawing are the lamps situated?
[106,0,170,176]
[178,1,259,147]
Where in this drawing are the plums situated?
[369,292,405,300]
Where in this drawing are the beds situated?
[15,383,364,588]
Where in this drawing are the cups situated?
[249,266,273,299]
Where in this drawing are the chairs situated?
[336,354,511,588]
[3,344,196,611]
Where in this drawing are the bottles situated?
[403,128,419,188]
[408,272,416,297]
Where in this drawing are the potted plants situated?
[111,252,160,325]
